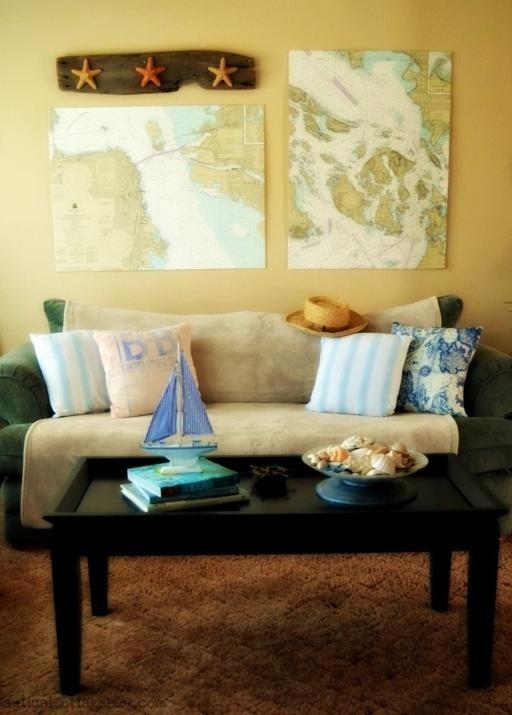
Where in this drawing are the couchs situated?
[1,295,512,556]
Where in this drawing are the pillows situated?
[305,321,484,418]
[28,321,202,419]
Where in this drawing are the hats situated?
[285,296,368,338]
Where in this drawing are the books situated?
[130,481,241,506]
[126,455,242,498]
[116,483,252,515]
